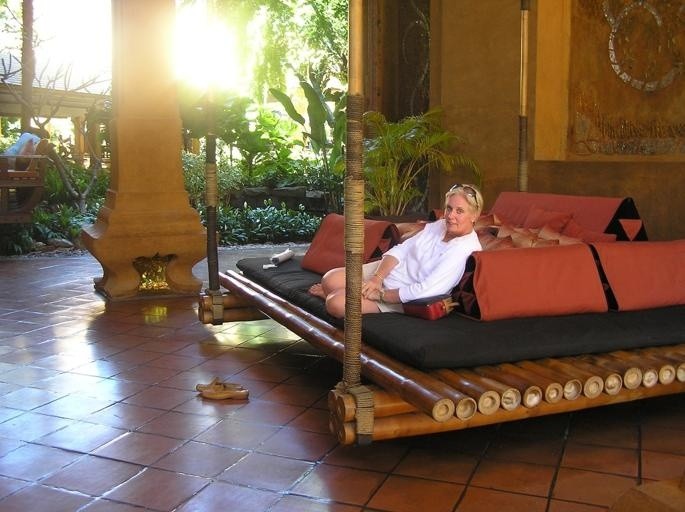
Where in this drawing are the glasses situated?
[451,184,478,210]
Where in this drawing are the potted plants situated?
[333,106,483,259]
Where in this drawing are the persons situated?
[308,184,483,319]
[5,132,48,200]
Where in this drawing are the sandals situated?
[196,377,249,399]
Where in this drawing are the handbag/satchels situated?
[404,293,460,319]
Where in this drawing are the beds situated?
[237,189,685,373]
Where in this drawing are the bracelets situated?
[375,271,384,279]
[379,289,384,303]
